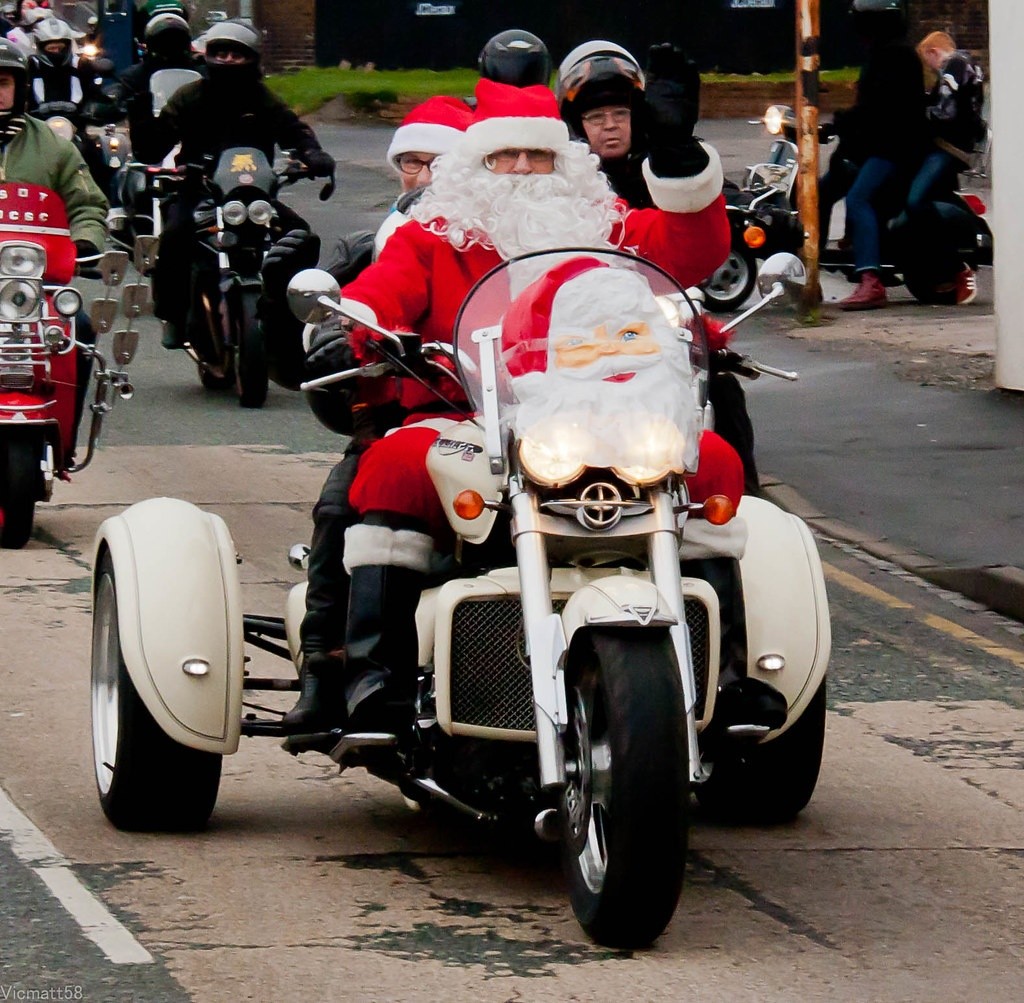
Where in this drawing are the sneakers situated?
[956,262,977,304]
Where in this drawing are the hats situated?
[387,96,475,175]
[466,77,570,155]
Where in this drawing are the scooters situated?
[689,103,991,316]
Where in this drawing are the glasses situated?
[217,46,243,60]
[581,108,632,125]
[492,148,554,163]
[395,155,436,174]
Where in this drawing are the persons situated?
[816,0,985,312]
[0,0,336,468]
[256,29,763,725]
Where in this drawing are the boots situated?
[342,512,436,734]
[680,511,790,732]
[283,633,349,732]
[838,268,887,309]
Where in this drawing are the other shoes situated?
[161,318,183,349]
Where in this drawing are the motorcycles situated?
[0,182,148,551]
[86,245,838,951]
[19,38,176,276]
[128,146,337,406]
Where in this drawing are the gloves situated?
[301,145,336,177]
[307,315,360,393]
[73,239,99,268]
[262,229,321,304]
[127,92,153,125]
[631,42,709,178]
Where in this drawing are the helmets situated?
[143,13,193,62]
[0,4,73,113]
[203,20,263,66]
[481,30,548,84]
[556,40,645,157]
[851,0,908,44]
